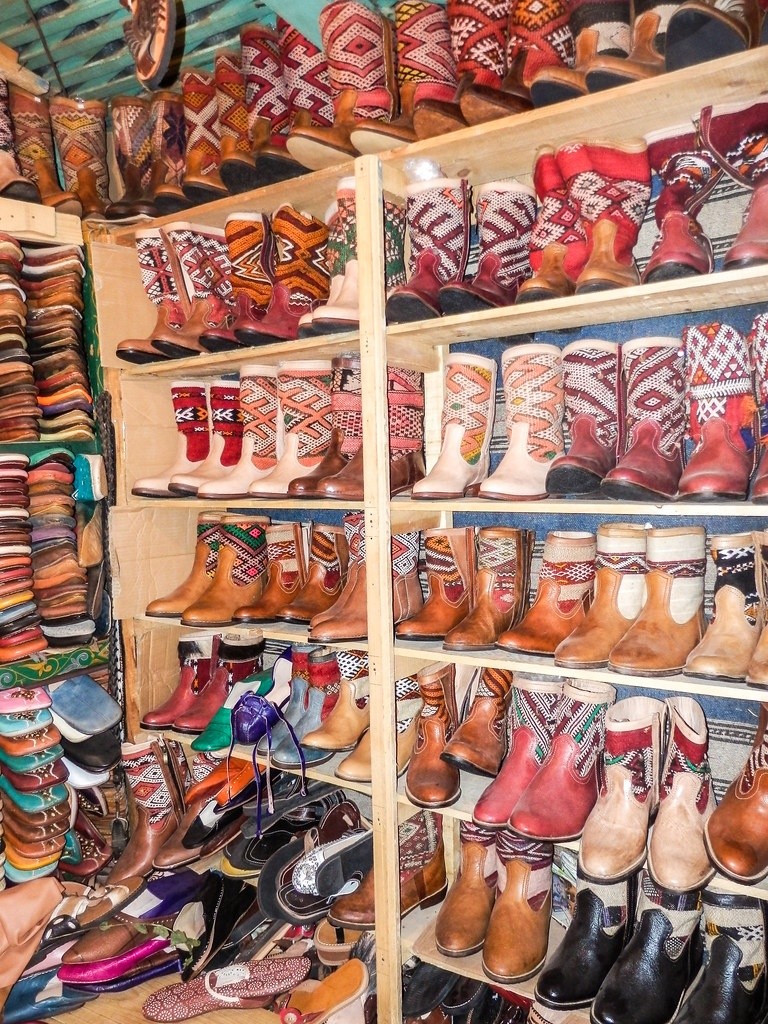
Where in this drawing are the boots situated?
[0,0,768,1024]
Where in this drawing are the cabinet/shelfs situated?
[1,41,768,1024]
